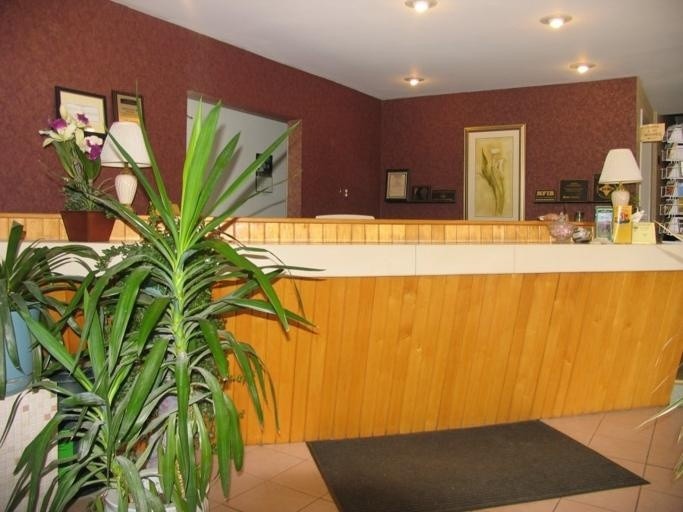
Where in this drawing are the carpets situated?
[306,418,650,512]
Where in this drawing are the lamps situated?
[598,147,643,207]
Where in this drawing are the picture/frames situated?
[462,122,526,220]
[384,168,408,201]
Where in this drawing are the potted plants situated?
[0,76,327,512]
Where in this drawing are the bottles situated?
[575,212,584,221]
